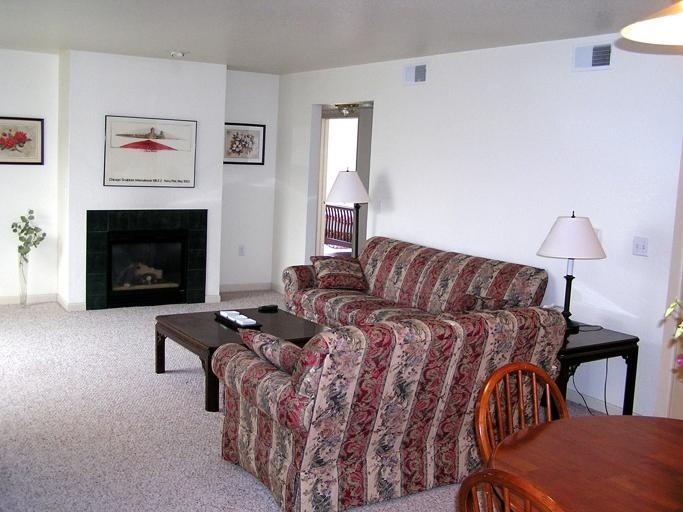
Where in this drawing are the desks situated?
[533,315,641,423]
[487,403,683,512]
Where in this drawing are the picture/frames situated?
[98,113,197,190]
[222,120,265,166]
[0,115,44,167]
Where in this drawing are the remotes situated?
[258,305,278,311]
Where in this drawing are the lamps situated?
[616,1,683,50]
[528,206,609,338]
[320,167,371,260]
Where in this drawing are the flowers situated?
[664,295,683,385]
[225,130,257,158]
[0,125,31,153]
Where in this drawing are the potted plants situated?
[2,204,47,306]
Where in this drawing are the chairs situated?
[448,464,566,512]
[469,358,574,512]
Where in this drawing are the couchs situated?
[201,304,572,512]
[276,226,549,337]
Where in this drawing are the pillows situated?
[305,251,371,294]
[230,322,305,376]
